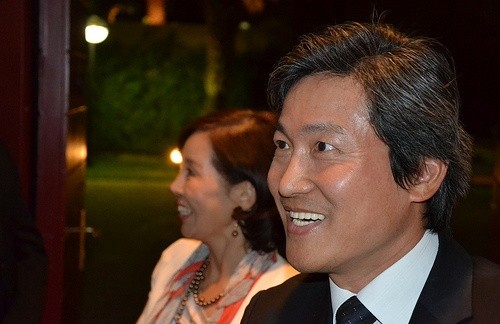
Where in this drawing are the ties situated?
[336,296,377,324]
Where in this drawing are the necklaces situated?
[174,255,226,324]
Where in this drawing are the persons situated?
[136,106,302,324]
[240,22,500,324]
